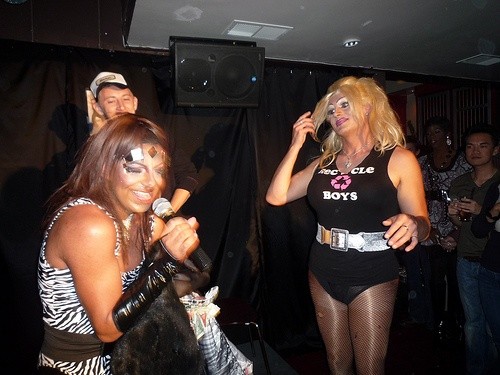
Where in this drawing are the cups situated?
[457,195,472,222]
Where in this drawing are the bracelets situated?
[486,212,499,221]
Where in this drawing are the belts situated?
[316,223,391,252]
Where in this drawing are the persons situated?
[265,77,430,375]
[37,72,220,375]
[402,116,500,375]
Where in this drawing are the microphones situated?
[152,198,212,272]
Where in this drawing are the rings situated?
[298,120,303,123]
[411,236,418,239]
[402,225,408,231]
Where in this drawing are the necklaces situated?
[121,213,133,242]
[340,146,364,169]
[441,149,451,167]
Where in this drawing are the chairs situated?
[217,296,271,375]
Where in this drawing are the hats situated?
[89,72,128,98]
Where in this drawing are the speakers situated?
[171,40,265,107]
